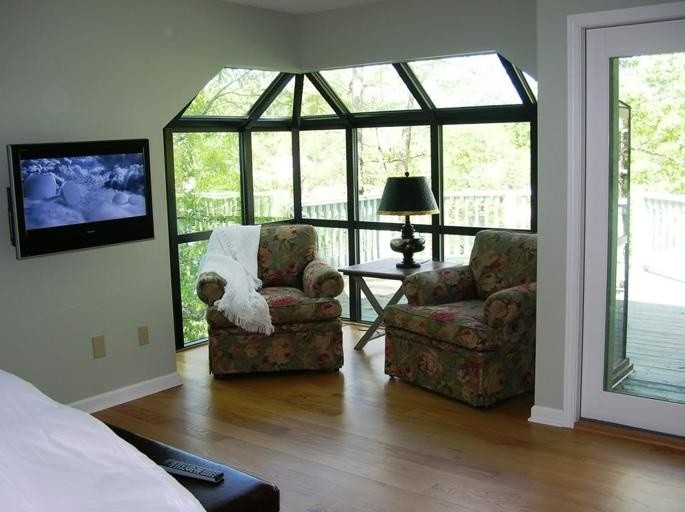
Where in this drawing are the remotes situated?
[156,453,226,485]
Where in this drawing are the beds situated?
[0,370,206,512]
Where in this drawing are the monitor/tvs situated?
[5,137,156,261]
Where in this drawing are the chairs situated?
[196,224,343,379]
[382,228,538,408]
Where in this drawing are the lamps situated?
[376,170,440,269]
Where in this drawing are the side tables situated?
[337,258,463,351]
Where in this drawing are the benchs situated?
[101,421,279,512]
